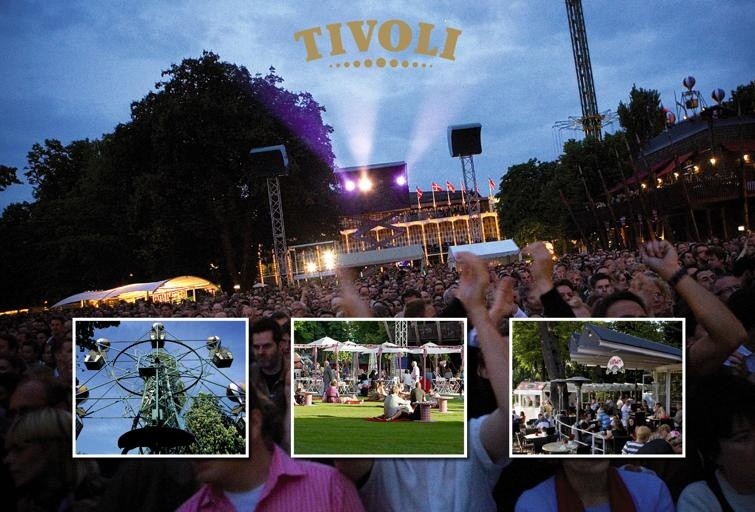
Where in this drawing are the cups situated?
[535,432,546,436]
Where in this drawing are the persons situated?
[0,168,754,511]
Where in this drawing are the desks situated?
[433,396,453,412]
[523,414,665,454]
[411,401,436,422]
[300,391,318,406]
